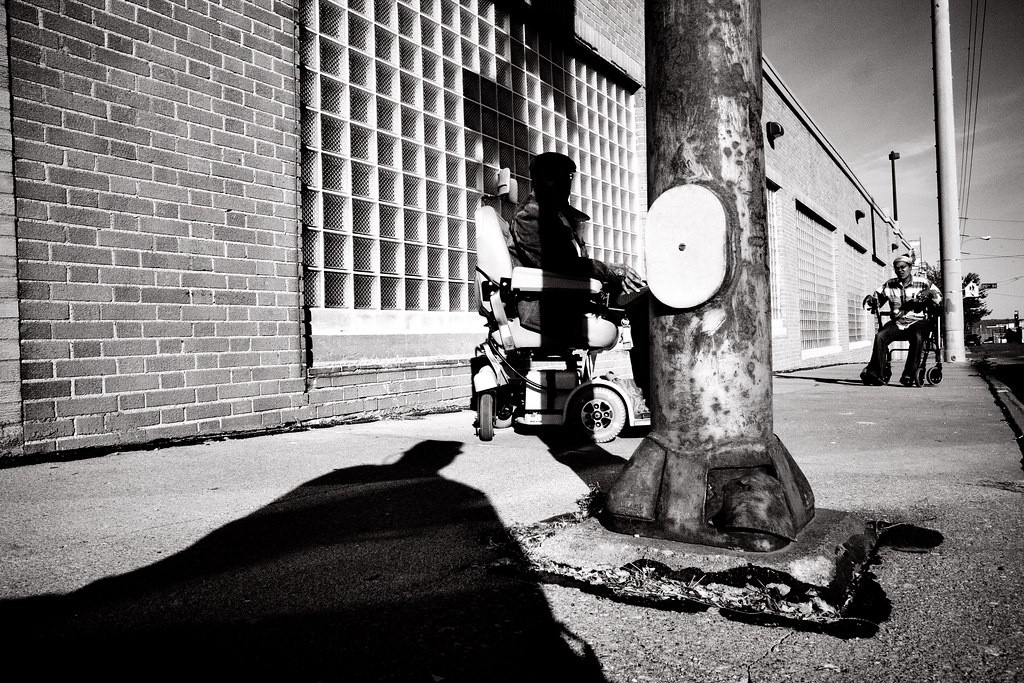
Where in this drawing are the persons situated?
[507,152,651,413]
[859,256,943,386]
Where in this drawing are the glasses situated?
[893,265,907,270]
[569,174,574,180]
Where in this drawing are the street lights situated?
[890,150,902,227]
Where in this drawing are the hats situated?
[529,152,576,173]
[893,253,912,265]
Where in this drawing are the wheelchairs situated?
[473,204,651,443]
[861,292,945,388]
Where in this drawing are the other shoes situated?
[900,375,913,386]
[860,371,883,386]
